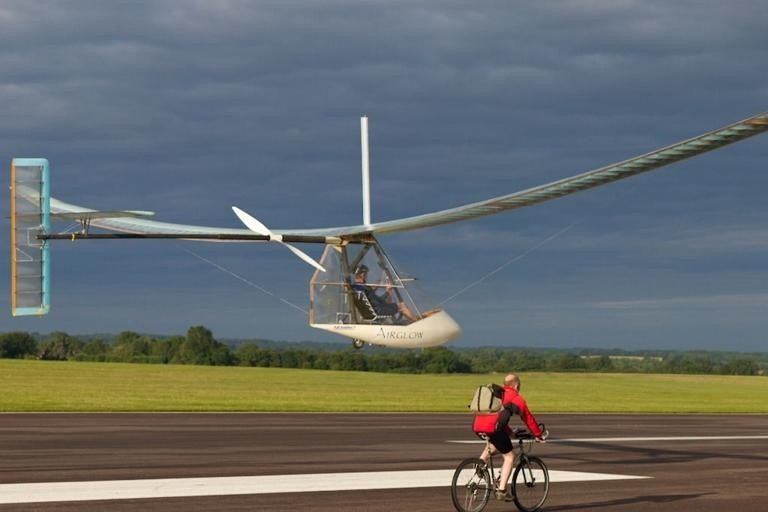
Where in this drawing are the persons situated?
[475,374,547,501]
[351,265,418,323]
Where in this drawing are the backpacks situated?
[468,383,505,415]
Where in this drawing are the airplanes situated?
[10,112,768,350]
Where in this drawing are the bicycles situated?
[451,423,549,512]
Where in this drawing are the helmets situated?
[352,264,369,274]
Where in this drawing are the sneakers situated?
[495,489,515,502]
